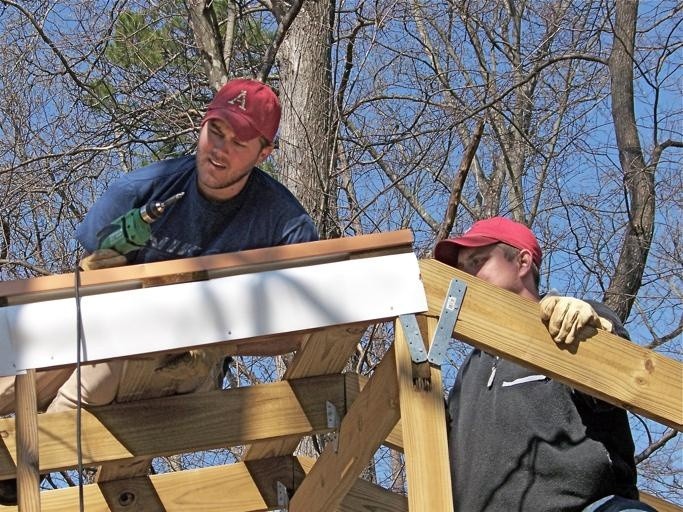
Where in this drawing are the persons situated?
[44,77,320,410]
[433,216,656,511]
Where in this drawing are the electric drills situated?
[96,192,186,256]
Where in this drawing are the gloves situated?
[538,295,614,346]
[75,246,128,273]
[152,341,239,381]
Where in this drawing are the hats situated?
[198,77,283,144]
[431,216,544,270]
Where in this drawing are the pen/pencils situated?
[487,356,499,388]
